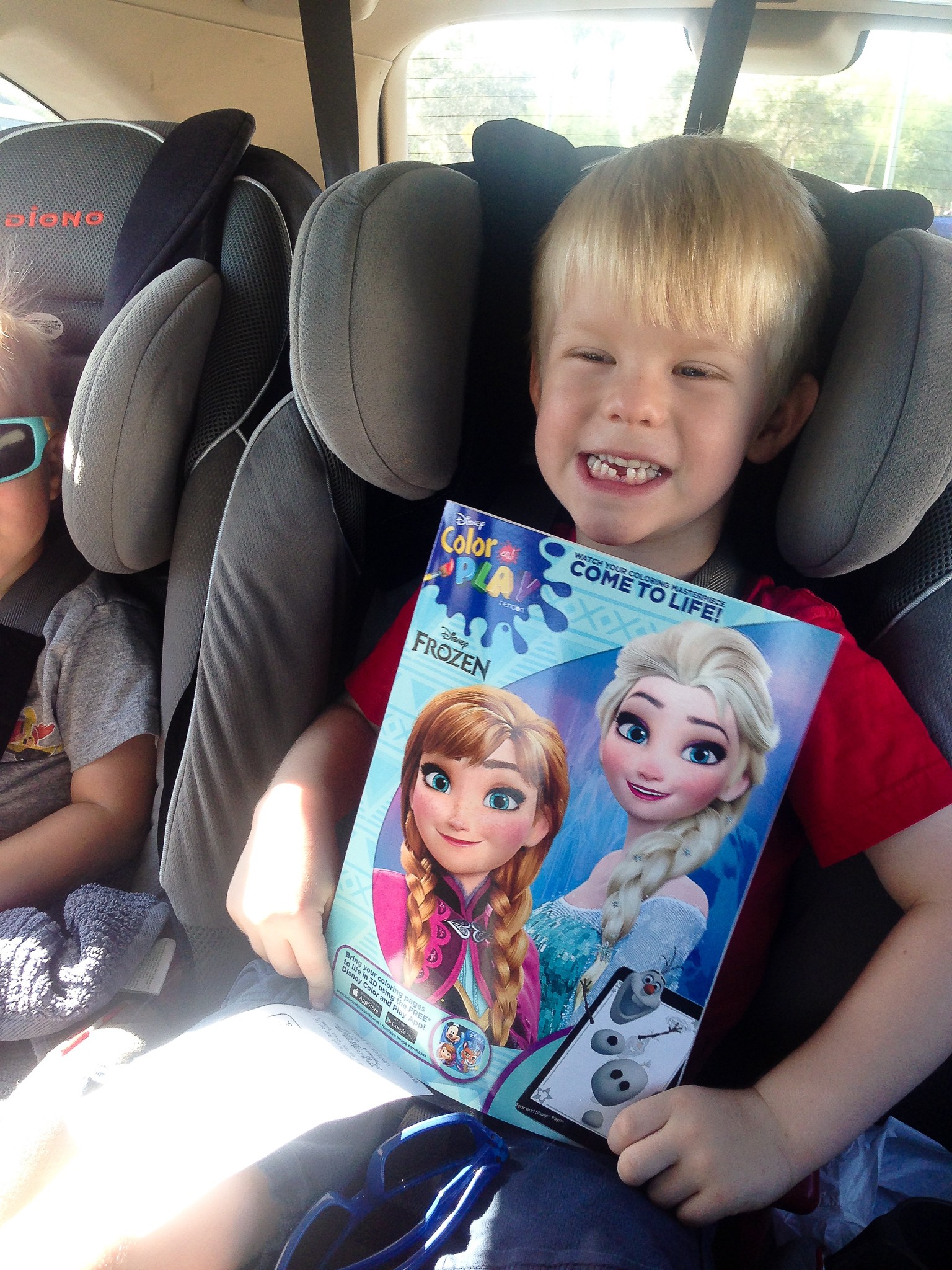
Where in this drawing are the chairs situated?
[0,101,950,1269]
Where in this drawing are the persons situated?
[2,130,951,1270]
[0,294,165,1102]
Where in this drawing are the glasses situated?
[0,415,62,485]
[273,1112,510,1270]
